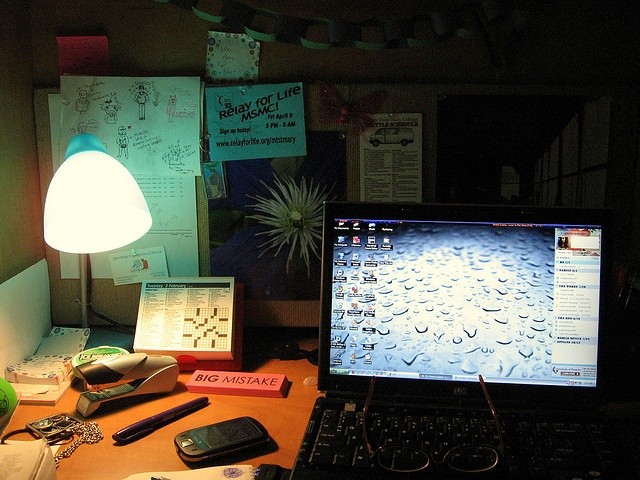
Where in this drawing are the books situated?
[4,325,91,384]
[0,436,57,480]
[7,367,78,406]
[121,463,255,480]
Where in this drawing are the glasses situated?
[362,374,504,473]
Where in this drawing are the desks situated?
[0,300,637,479]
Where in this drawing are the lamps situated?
[43,152,151,351]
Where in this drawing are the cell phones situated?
[173,416,271,463]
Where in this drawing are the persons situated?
[556,238,570,248]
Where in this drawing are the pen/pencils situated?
[112,396,209,442]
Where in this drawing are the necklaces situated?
[55,420,106,460]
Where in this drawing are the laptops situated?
[290,201,640,476]
